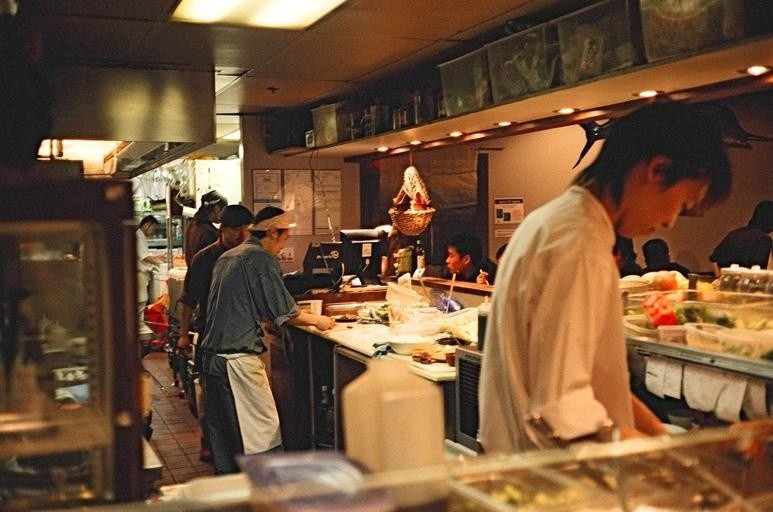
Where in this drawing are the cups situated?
[309,299,323,316]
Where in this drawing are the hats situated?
[217,205,253,227]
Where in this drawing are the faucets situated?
[429,289,445,300]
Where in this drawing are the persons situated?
[704,200,773,271]
[611,234,644,277]
[442,236,498,288]
[134,215,166,336]
[458,95,733,463]
[186,206,340,474]
[637,237,692,278]
[182,189,232,416]
[476,242,509,285]
[174,203,256,465]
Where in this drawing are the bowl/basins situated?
[356,309,370,318]
[387,334,435,356]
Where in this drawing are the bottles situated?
[718,263,773,295]
[477,295,491,352]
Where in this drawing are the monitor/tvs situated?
[341,228,389,287]
[303,242,344,287]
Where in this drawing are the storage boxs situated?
[639,1,752,63]
[551,1,640,83]
[484,21,557,104]
[437,46,493,116]
